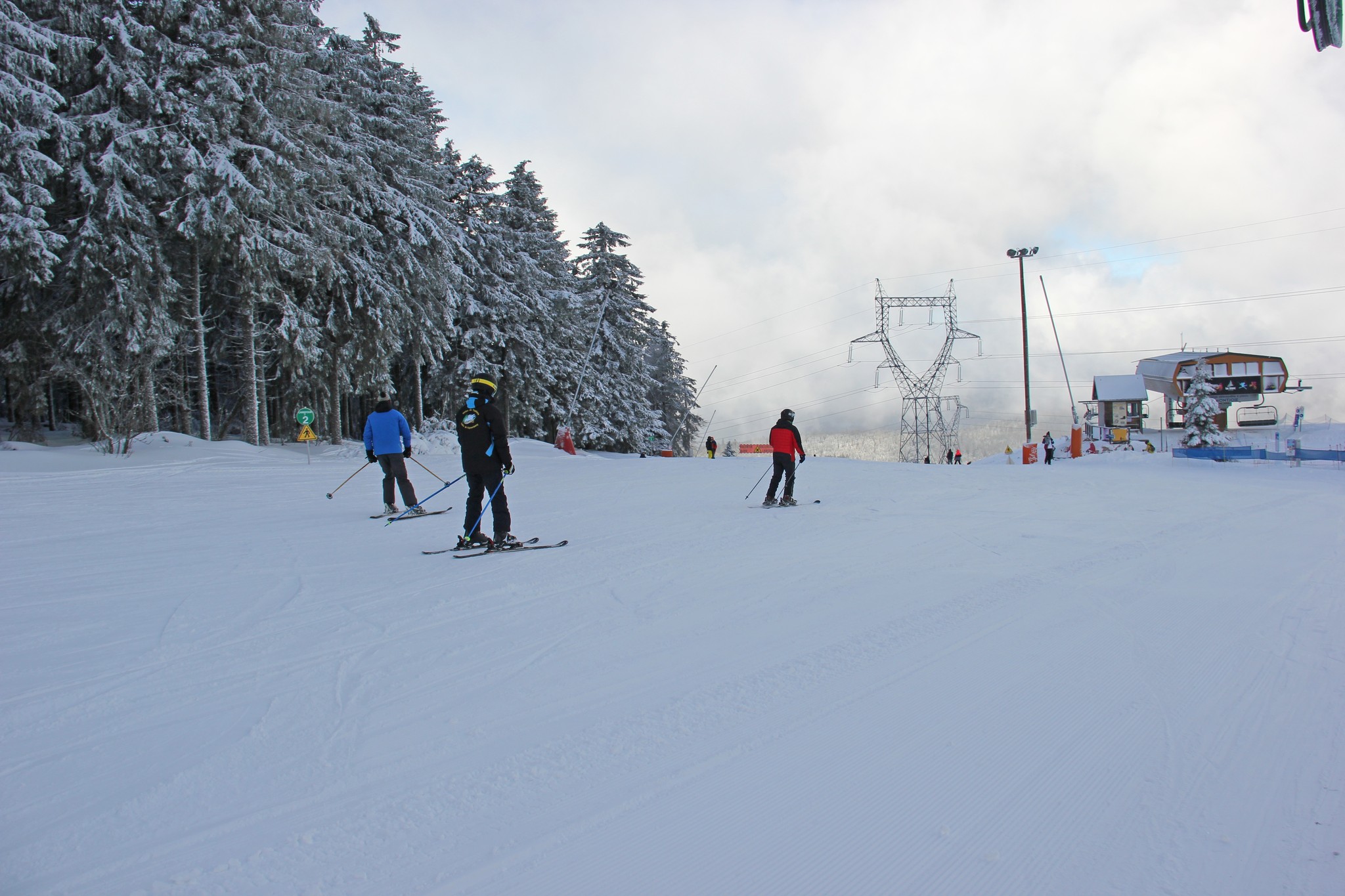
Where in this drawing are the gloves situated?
[364,450,377,464]
[502,462,515,476]
[401,445,411,459]
[799,454,807,463]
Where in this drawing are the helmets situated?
[780,407,796,425]
[469,372,499,397]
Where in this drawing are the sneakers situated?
[778,497,798,507]
[493,531,516,546]
[383,503,398,512]
[463,528,489,546]
[762,495,779,506]
[405,504,427,515]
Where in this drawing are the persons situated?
[1124,441,1134,451]
[1142,440,1155,453]
[710,437,717,459]
[363,390,427,517]
[1085,440,1096,454]
[640,451,646,458]
[1066,445,1071,453]
[705,436,713,459]
[453,372,524,552]
[813,454,816,457]
[924,456,930,464]
[946,449,953,464]
[761,408,806,506]
[953,449,961,464]
[1042,431,1055,465]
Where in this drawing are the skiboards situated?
[370,507,453,521]
[421,537,569,559]
[747,499,821,509]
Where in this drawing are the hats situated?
[376,392,391,403]
